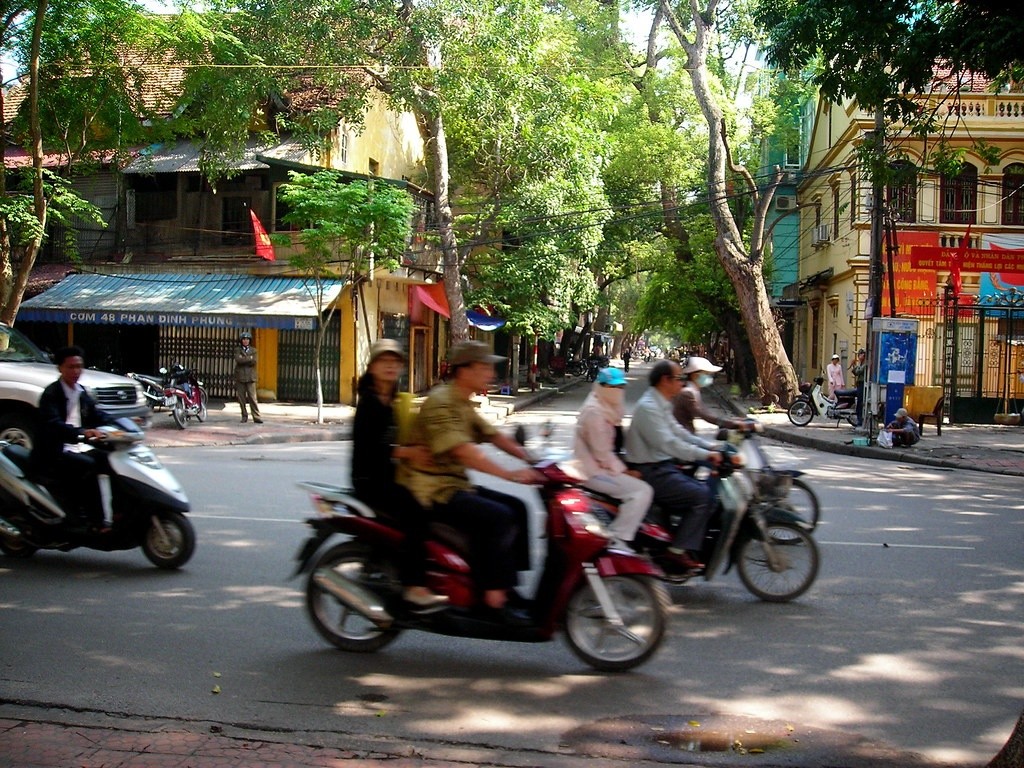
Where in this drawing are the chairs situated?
[918,396,945,436]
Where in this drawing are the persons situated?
[665,345,688,361]
[27,346,139,533]
[850,348,866,427]
[886,408,920,448]
[643,346,651,358]
[827,354,845,400]
[625,359,723,570]
[556,368,654,555]
[623,349,631,373]
[351,336,449,605]
[592,342,603,355]
[401,340,549,620]
[234,332,263,423]
[671,357,763,435]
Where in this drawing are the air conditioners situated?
[775,194,797,210]
[811,224,828,244]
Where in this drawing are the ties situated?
[244,346,248,353]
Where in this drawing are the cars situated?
[0,323,154,433]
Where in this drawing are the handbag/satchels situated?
[876,429,894,449]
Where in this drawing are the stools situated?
[501,385,511,395]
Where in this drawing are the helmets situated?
[239,332,252,345]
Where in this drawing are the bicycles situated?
[624,360,630,372]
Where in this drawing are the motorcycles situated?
[548,412,822,605]
[289,415,668,672]
[788,369,861,428]
[126,359,208,429]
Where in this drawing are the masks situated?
[693,372,713,388]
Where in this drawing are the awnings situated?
[16,274,350,332]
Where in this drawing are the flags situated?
[251,210,276,261]
[949,226,971,294]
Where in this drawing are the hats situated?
[370,338,405,359]
[855,348,866,354]
[449,339,508,365]
[596,367,628,386]
[894,408,907,418]
[683,357,724,374]
[832,354,840,359]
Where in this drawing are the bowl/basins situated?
[852,437,870,446]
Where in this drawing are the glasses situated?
[673,374,688,383]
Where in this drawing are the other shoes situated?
[240,416,248,422]
[255,419,263,423]
[479,598,534,623]
[403,590,449,614]
[605,539,647,557]
[665,549,706,568]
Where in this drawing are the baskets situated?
[746,466,793,501]
[798,381,812,393]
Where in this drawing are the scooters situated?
[549,346,610,382]
[0,412,199,572]
